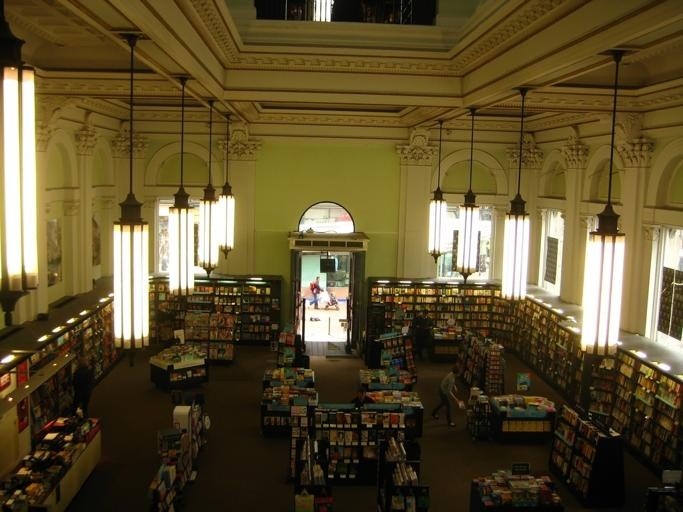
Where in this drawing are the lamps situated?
[426,87,531,313]
[114,37,148,350]
[218,116,237,261]
[196,99,219,276]
[170,81,195,296]
[581,52,627,372]
[0,0,39,326]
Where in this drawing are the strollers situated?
[322,289,339,311]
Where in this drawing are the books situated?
[361,336,422,408]
[494,290,584,404]
[373,285,493,354]
[550,409,603,495]
[476,471,561,509]
[459,331,503,437]
[590,345,683,463]
[149,409,206,511]
[261,367,423,511]
[2,415,97,511]
[149,279,298,380]
[28,304,113,428]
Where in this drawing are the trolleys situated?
[466,401,495,443]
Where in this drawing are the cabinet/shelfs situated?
[287,398,431,512]
[363,332,424,442]
[261,326,318,434]
[182,309,235,366]
[467,463,572,510]
[152,399,211,512]
[488,275,682,512]
[431,319,506,396]
[148,345,204,392]
[365,275,489,351]
[157,277,282,349]
[2,275,155,510]
[468,386,490,444]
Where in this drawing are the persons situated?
[433,365,461,426]
[330,293,337,304]
[310,276,324,309]
